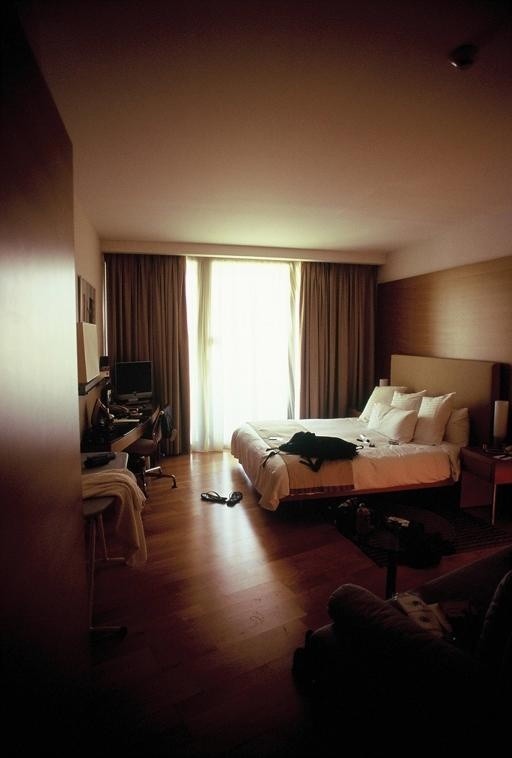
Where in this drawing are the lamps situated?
[493,399,509,447]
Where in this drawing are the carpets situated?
[320,493,512,568]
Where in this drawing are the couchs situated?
[292,545,512,756]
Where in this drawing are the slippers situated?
[227,492,243,507]
[201,491,226,503]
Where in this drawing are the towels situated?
[81,468,147,568]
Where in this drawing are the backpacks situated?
[279,431,357,461]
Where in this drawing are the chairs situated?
[124,410,178,504]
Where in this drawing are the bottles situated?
[360,432,370,443]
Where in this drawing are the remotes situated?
[113,418,140,423]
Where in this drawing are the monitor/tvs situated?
[113,361,153,406]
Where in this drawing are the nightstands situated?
[459,447,512,525]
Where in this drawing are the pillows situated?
[325,582,478,703]
[357,384,471,448]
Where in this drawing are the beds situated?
[231,354,500,513]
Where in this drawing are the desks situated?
[82,398,161,490]
[80,451,129,636]
